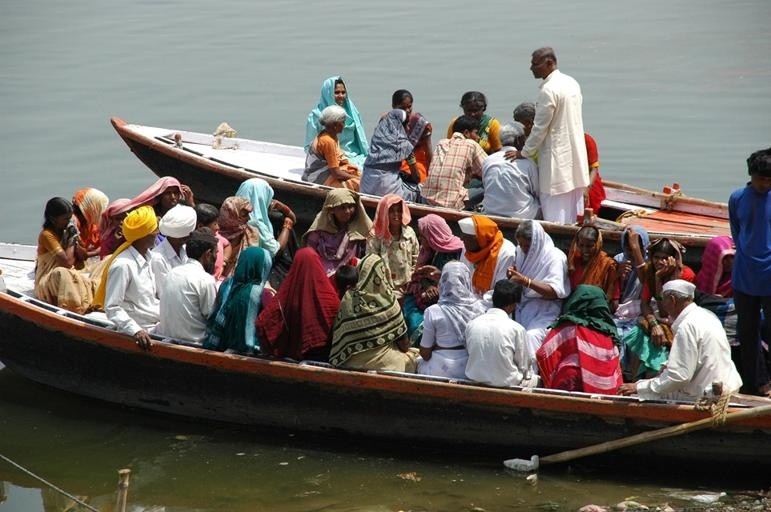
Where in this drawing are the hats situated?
[663,280,696,299]
[458,216,476,236]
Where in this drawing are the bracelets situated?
[528,279,531,289]
[648,320,658,327]
[282,220,292,231]
[654,293,664,301]
[67,264,75,272]
[634,262,646,269]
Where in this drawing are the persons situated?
[219,247,274,355]
[578,133,606,223]
[34,197,100,316]
[506,220,571,376]
[305,75,368,169]
[514,103,536,139]
[302,105,360,191]
[624,237,696,303]
[360,109,421,202]
[159,230,218,347]
[482,121,542,221]
[616,279,743,402]
[457,215,515,296]
[151,176,195,219]
[464,280,532,387]
[377,89,433,181]
[366,194,420,299]
[415,260,487,379]
[92,205,160,353]
[194,204,232,293]
[236,178,300,289]
[447,91,502,155]
[404,214,465,346]
[217,196,260,277]
[503,47,590,225]
[566,224,620,315]
[692,235,740,348]
[258,246,341,361]
[612,224,649,301]
[329,252,420,375]
[302,188,373,273]
[71,188,109,279]
[100,198,134,254]
[149,205,197,299]
[334,262,358,293]
[536,284,623,396]
[728,147,771,398]
[421,114,488,210]
